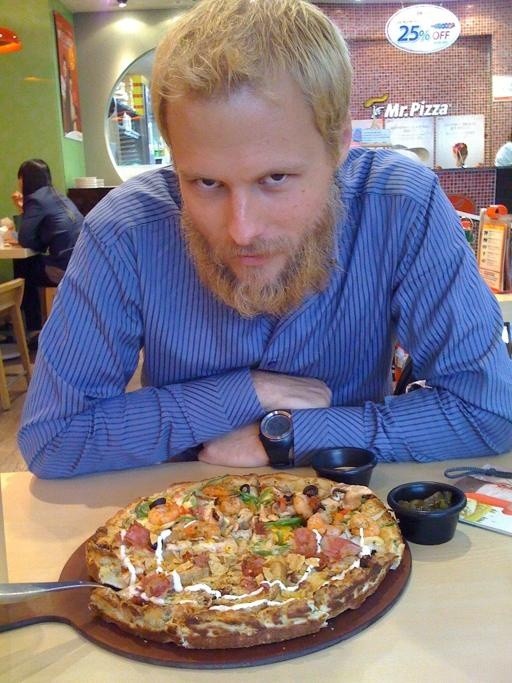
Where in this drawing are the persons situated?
[8,157,88,349]
[14,1,512,482]
[58,54,81,132]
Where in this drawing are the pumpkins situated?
[486,205,508,220]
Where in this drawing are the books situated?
[448,461,512,536]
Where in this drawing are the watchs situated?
[254,405,295,469]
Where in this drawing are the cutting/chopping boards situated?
[0,474,414,671]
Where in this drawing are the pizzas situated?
[85,472,406,649]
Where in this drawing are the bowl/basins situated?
[309,445,379,490]
[385,479,466,547]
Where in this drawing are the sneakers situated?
[2,319,41,350]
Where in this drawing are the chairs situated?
[0,276,32,410]
